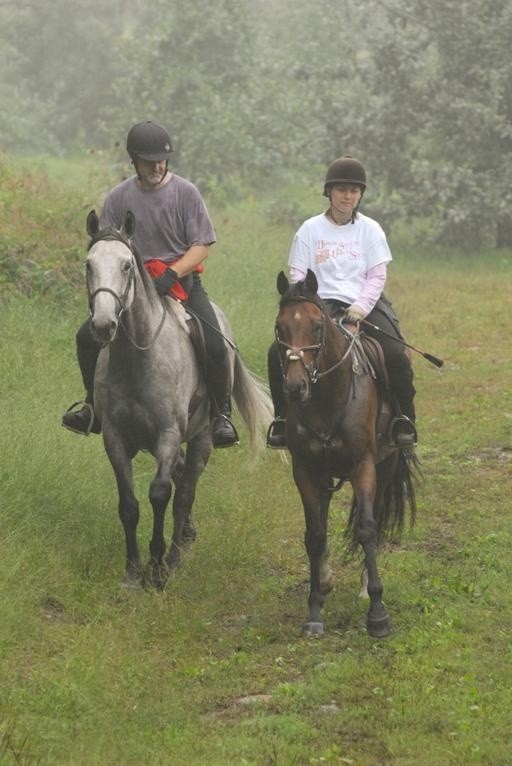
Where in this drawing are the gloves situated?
[153,266,180,299]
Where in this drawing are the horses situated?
[85,209,290,598]
[275,268,429,639]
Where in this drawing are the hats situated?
[128,119,174,162]
[325,152,371,189]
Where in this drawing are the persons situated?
[63,120,239,446]
[269,155,416,446]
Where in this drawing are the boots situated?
[268,419,289,448]
[391,416,418,446]
[61,398,105,436]
[206,388,238,447]
